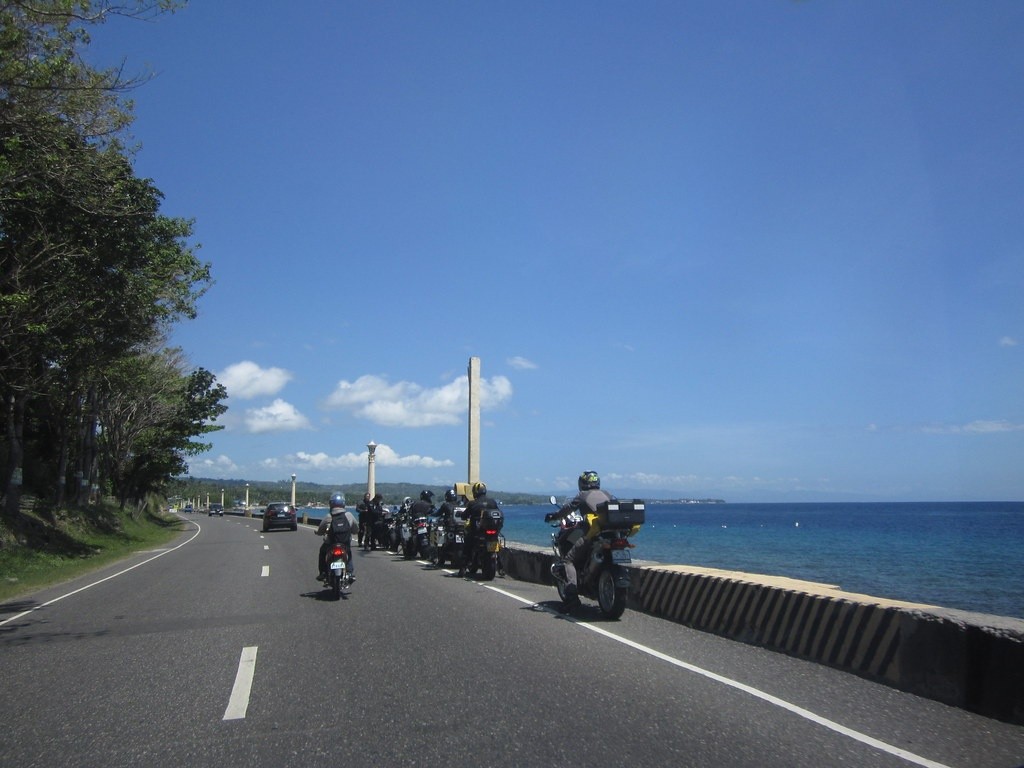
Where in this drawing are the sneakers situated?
[560,598,581,614]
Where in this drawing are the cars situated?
[184,504,193,513]
[260,502,300,532]
[208,503,224,516]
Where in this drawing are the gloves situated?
[544,512,557,523]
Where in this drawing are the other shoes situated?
[358,538,362,546]
[316,569,326,580]
[457,568,467,577]
[497,560,506,577]
[435,559,446,568]
[346,572,357,581]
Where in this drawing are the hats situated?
[375,494,382,500]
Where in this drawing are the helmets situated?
[578,470,600,491]
[445,489,456,502]
[420,490,435,503]
[472,482,487,498]
[329,491,346,511]
[402,496,411,504]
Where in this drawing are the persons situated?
[545,470,618,598]
[409,489,437,521]
[356,493,388,551]
[315,493,359,581]
[456,483,507,577]
[392,506,399,516]
[430,488,463,568]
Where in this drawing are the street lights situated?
[221,488,225,507]
[192,497,196,508]
[291,473,297,508]
[206,492,210,508]
[245,483,250,507]
[197,494,201,508]
[366,440,378,501]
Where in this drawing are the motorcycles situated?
[454,499,504,581]
[320,527,354,601]
[375,501,470,569]
[549,495,646,620]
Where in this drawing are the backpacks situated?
[329,512,351,544]
